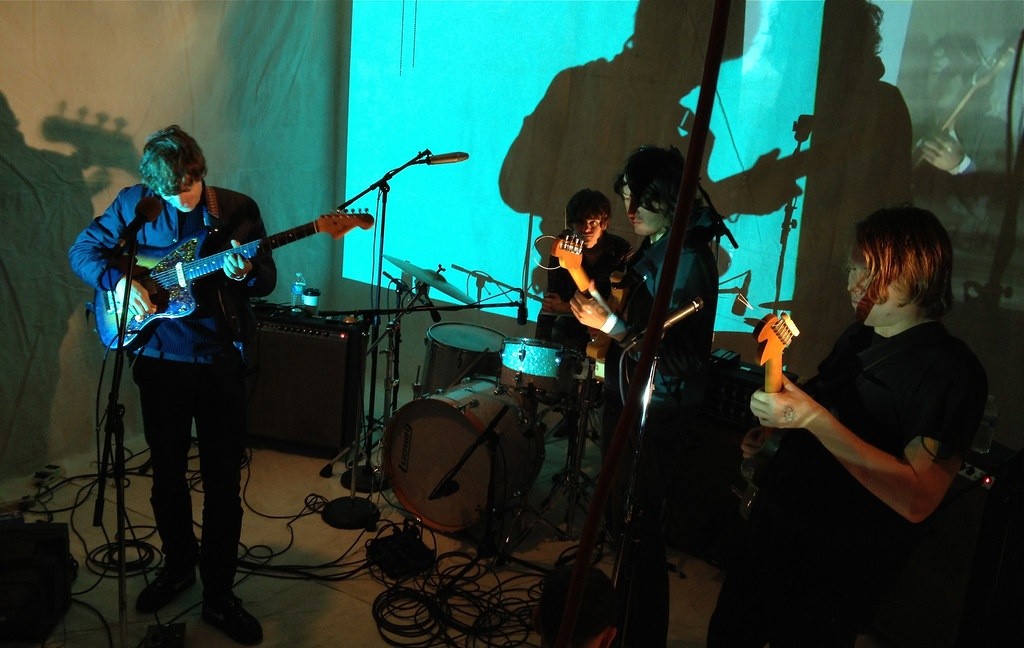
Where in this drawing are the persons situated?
[542,189,631,315]
[706,206,989,648]
[532,565,617,648]
[68,124,277,646]
[571,147,719,648]
[914,35,1005,224]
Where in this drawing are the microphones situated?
[417,153,469,165]
[107,198,163,269]
[517,290,527,325]
[428,480,459,500]
[627,296,705,351]
[424,292,441,323]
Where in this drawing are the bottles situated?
[291,272,306,305]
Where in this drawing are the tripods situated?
[428,402,552,600]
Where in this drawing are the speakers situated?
[860,437,1024,648]
[0,522,72,644]
[656,409,760,567]
[239,304,370,459]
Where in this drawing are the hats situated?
[540,563,618,640]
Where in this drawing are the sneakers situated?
[201,586,264,646]
[135,562,197,614]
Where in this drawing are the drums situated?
[418,322,509,395]
[379,377,544,534]
[500,337,564,378]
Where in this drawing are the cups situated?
[303,288,322,315]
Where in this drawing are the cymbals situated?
[759,300,854,310]
[382,254,477,306]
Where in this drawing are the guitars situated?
[552,229,632,364]
[739,312,800,526]
[93,208,374,348]
[907,34,1022,229]
